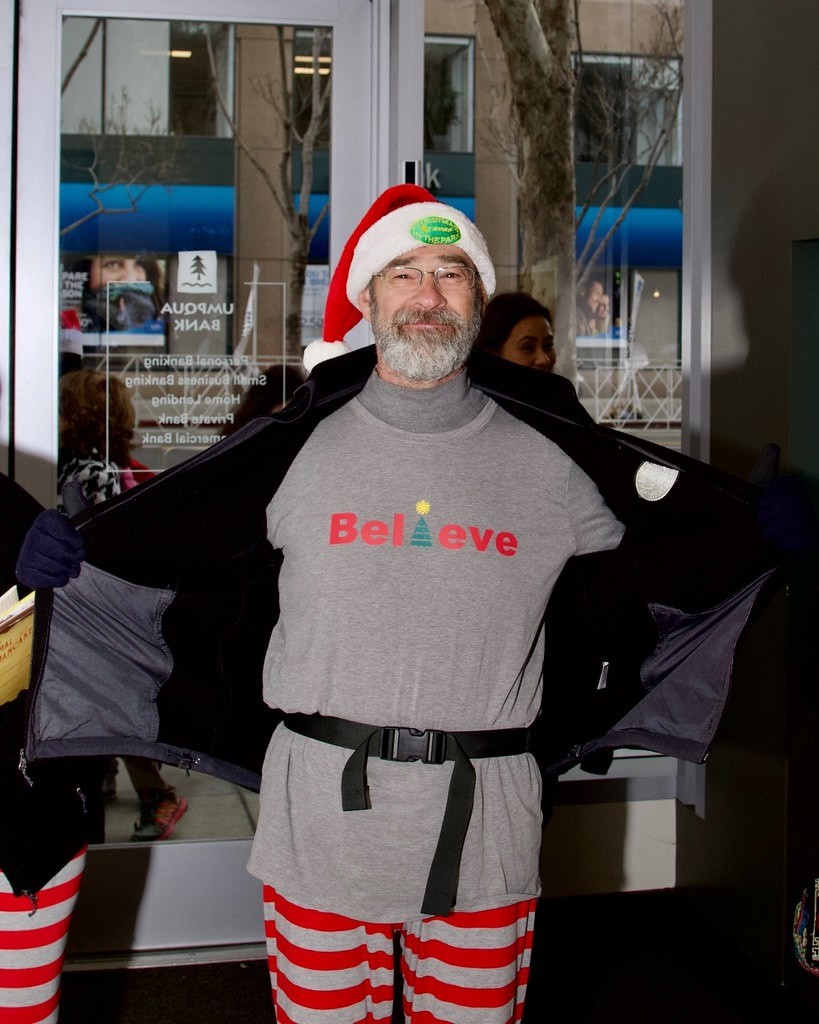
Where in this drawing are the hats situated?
[303,183,496,373]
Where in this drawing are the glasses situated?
[374,266,479,291]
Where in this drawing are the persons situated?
[0,472,92,1023]
[577,278,611,338]
[219,364,308,440]
[59,293,170,371]
[16,181,819,1024]
[56,370,190,841]
[64,251,157,297]
[478,289,561,374]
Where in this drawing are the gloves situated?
[749,443,819,570]
[17,508,86,590]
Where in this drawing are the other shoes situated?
[130,790,188,841]
[102,781,116,801]
